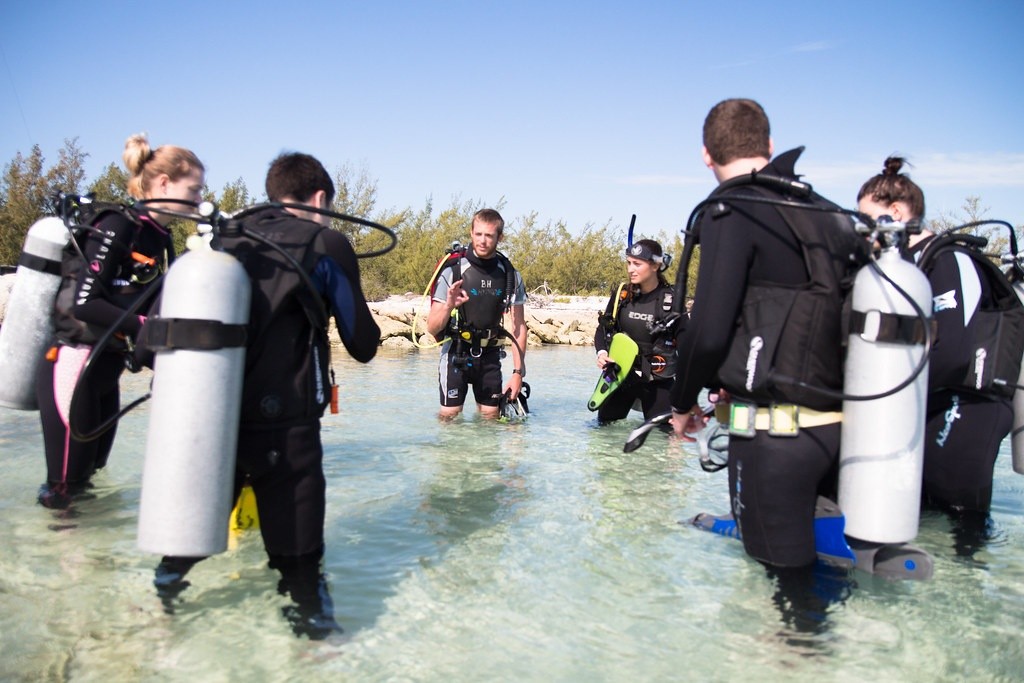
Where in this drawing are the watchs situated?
[513,369,525,377]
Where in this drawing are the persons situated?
[671,98,873,565]
[35,136,205,481]
[428,209,528,415]
[858,155,1024,514]
[182,152,381,557]
[594,239,688,422]
